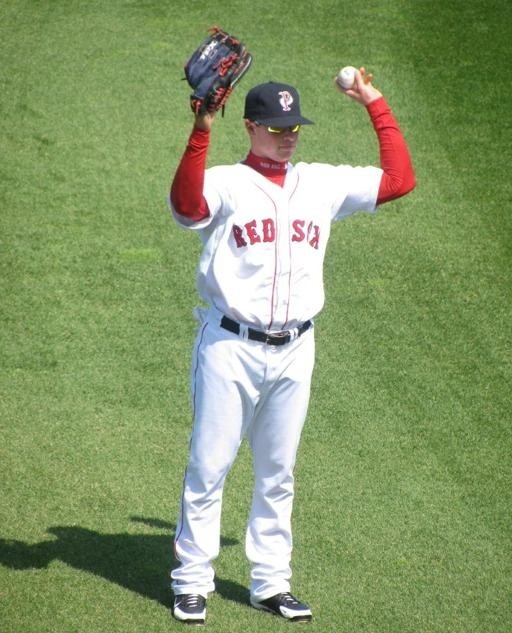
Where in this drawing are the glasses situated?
[255,121,301,135]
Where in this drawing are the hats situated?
[243,81,314,130]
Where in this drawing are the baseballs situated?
[338,65,358,89]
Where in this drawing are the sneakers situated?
[250,591,313,624]
[174,594,207,624]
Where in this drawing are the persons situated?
[169,66,417,622]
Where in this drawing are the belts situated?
[220,315,311,346]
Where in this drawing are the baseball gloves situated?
[184,30,252,112]
